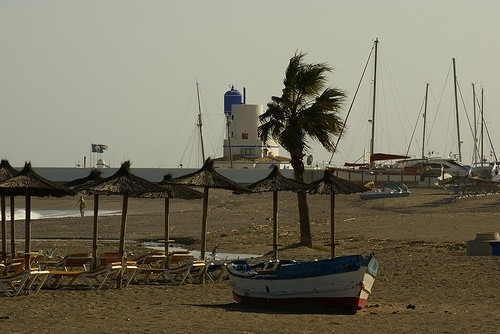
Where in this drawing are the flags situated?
[92,144,106,154]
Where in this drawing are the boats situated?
[225,252,380,311]
[359,181,411,200]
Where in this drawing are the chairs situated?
[0,250,241,297]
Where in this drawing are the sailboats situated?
[179,36,500,194]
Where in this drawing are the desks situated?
[466,238,500,256]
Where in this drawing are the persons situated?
[78,196,84,218]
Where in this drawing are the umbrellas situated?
[292,169,373,258]
[126,175,206,256]
[0,159,20,255]
[54,170,116,257]
[74,161,173,254]
[0,162,70,270]
[159,156,248,261]
[233,166,317,262]
